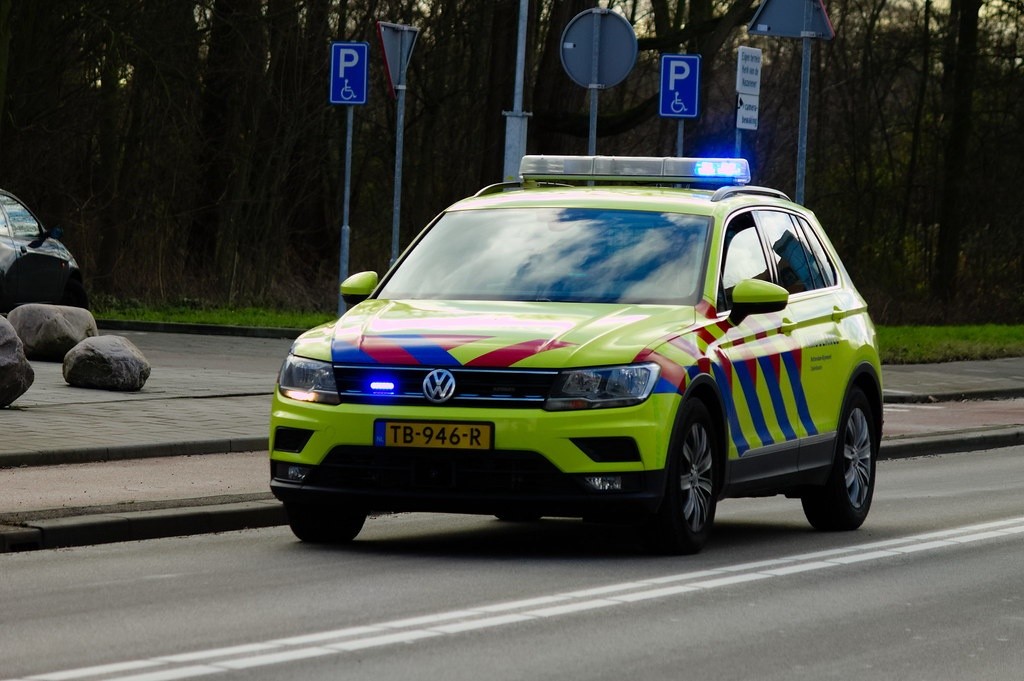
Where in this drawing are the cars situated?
[0,189,89,313]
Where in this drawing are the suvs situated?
[268,156,884,556]
